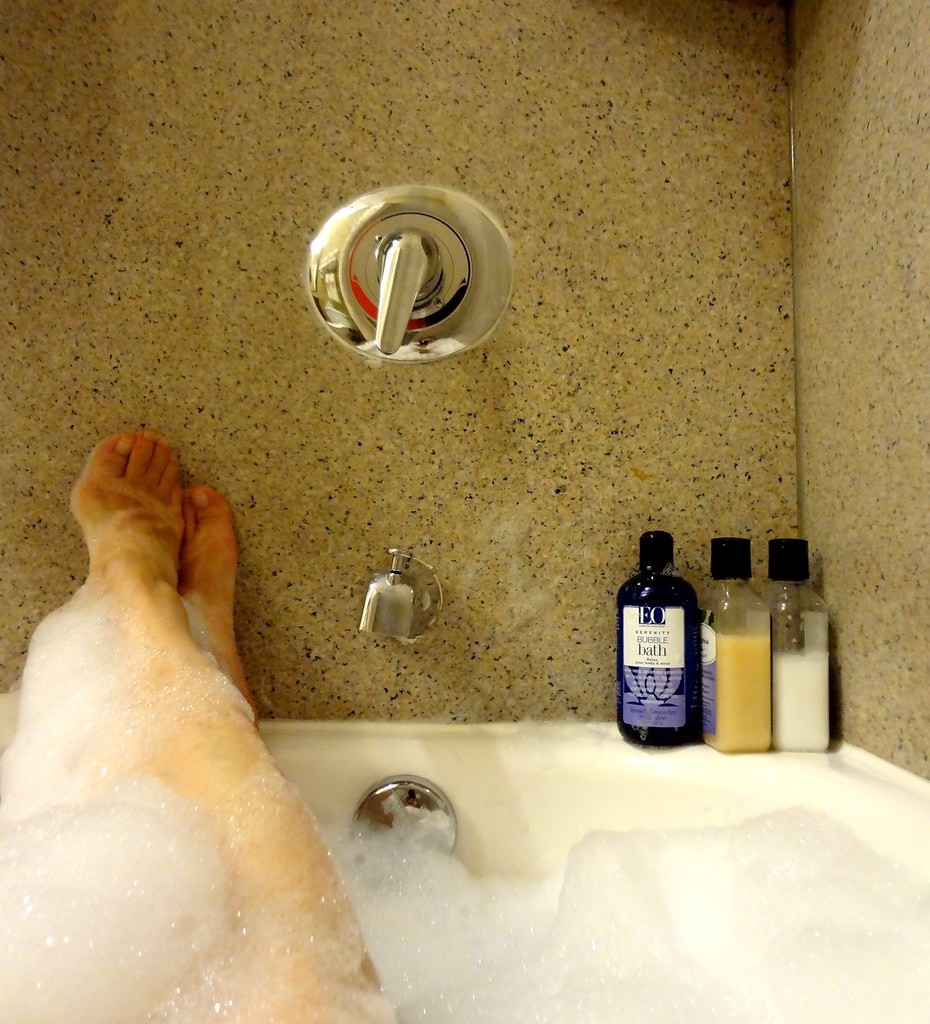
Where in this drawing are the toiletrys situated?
[615,529,830,752]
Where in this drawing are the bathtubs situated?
[6,718,930,1024]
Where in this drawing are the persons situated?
[0,426,389,1024]
